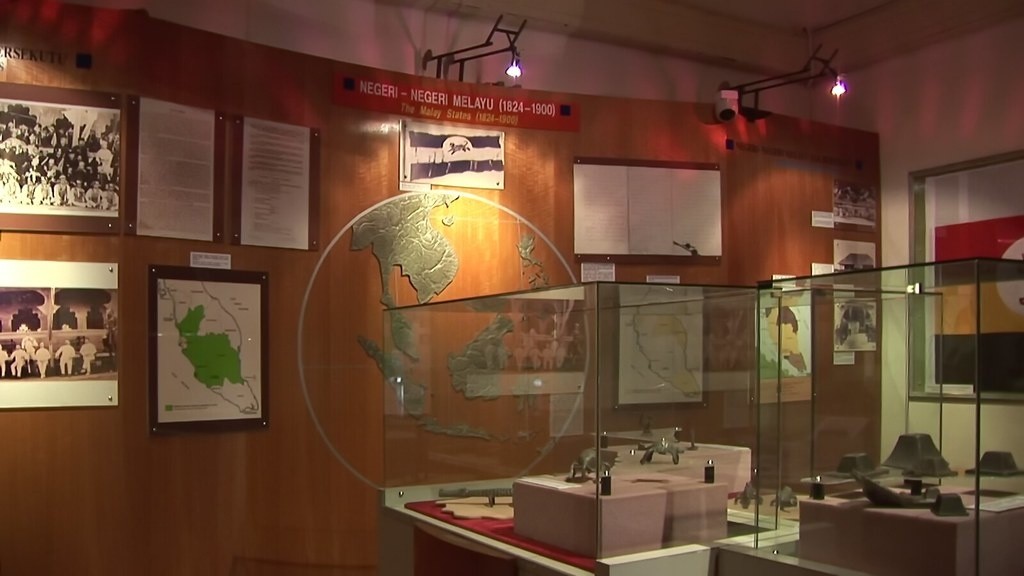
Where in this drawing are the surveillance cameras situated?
[717,90,740,120]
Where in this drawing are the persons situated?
[79,338,97,376]
[0,344,9,377]
[0,115,119,212]
[21,330,38,374]
[36,342,51,379]
[55,339,75,376]
[9,344,29,377]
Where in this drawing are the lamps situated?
[431,14,527,82]
[732,43,846,109]
